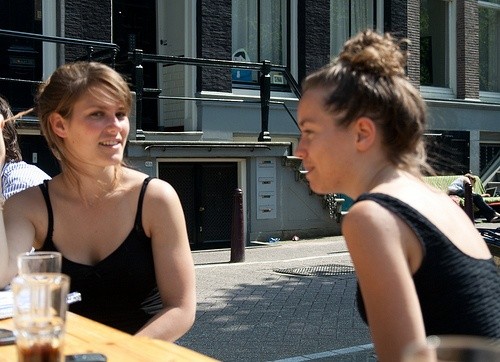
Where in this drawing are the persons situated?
[297,29,500,362]
[448,173,500,222]
[1,62,196,344]
[1,98,53,291]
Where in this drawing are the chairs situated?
[422,175,500,206]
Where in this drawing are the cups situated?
[12,273,69,362]
[18,252,61,274]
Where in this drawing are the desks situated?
[0,310,225,362]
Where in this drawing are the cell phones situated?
[0,329,16,345]
[65,353,107,362]
